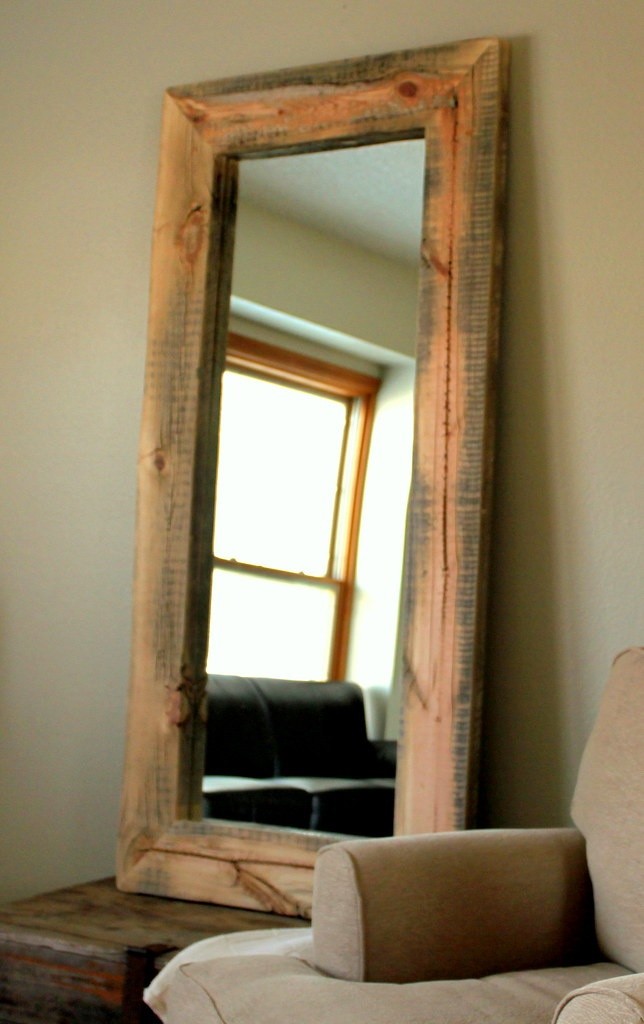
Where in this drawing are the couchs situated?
[204,670,398,841]
[142,641,644,1024]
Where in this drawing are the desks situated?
[0,875,306,1024]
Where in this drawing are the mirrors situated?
[116,37,511,915]
